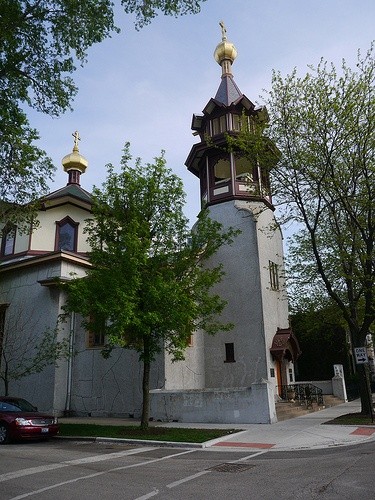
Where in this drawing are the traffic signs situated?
[353,346,369,365]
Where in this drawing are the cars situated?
[0,396,60,445]
